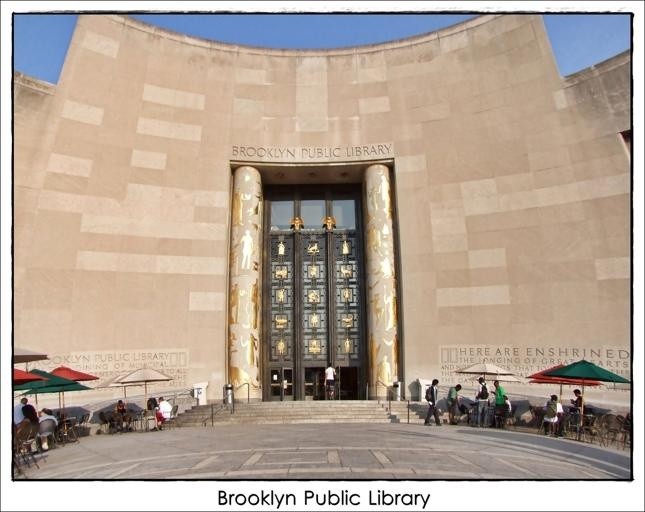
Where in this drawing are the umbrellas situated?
[543,360,630,402]
[13,347,49,373]
[471,373,522,383]
[524,364,605,385]
[453,362,517,376]
[529,379,603,401]
[13,365,174,415]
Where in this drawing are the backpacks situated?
[547,402,556,418]
[426,387,433,400]
[480,383,487,399]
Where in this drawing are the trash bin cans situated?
[393,382,402,401]
[223,384,234,404]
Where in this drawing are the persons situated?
[14,398,63,451]
[114,396,175,432]
[490,380,506,428]
[322,362,339,400]
[475,377,490,428]
[542,388,587,436]
[423,378,442,426]
[503,395,512,420]
[446,384,464,426]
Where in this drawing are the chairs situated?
[529,406,630,451]
[14,416,77,475]
[457,401,517,429]
[98,405,178,432]
[78,413,90,430]
